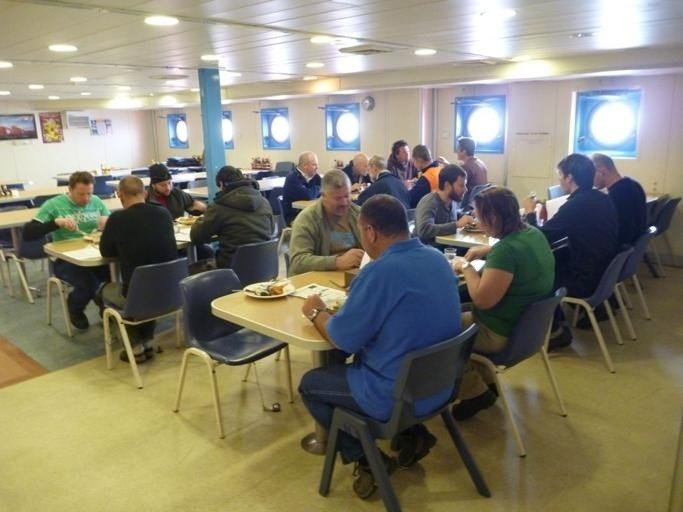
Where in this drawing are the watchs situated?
[307,305,327,322]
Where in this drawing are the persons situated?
[145,164,208,220]
[523,154,618,353]
[298,193,463,500]
[98,176,180,363]
[451,186,555,422]
[589,153,648,310]
[281,137,488,278]
[22,171,112,330]
[190,166,275,268]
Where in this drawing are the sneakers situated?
[352,450,396,500]
[68,298,90,331]
[452,389,497,422]
[397,425,439,467]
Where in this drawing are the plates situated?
[463,226,484,233]
[82,233,101,244]
[244,281,295,299]
[175,216,201,225]
[451,255,468,278]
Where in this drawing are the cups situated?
[444,247,457,265]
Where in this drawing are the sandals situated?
[119,348,155,364]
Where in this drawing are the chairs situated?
[642,196,677,278]
[173,269,296,440]
[0,155,423,390]
[548,247,632,372]
[578,226,655,341]
[443,286,569,457]
[318,322,485,511]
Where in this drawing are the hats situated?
[149,163,173,183]
